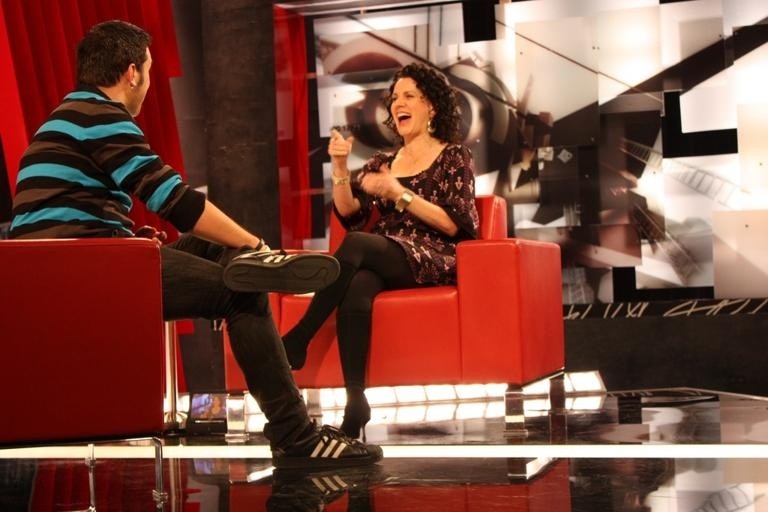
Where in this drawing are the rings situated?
[377,182,382,189]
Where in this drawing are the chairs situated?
[281,196,567,438]
[2,234,165,501]
[164,318,226,436]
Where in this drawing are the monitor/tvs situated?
[188,390,230,423]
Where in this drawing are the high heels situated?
[339,384,370,443]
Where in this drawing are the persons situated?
[8,21,383,469]
[264,464,380,511]
[282,63,479,443]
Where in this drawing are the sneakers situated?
[269,421,384,470]
[222,244,341,296]
[272,466,383,506]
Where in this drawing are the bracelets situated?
[253,238,265,250]
[266,245,271,253]
[395,191,413,212]
[332,170,352,184]
